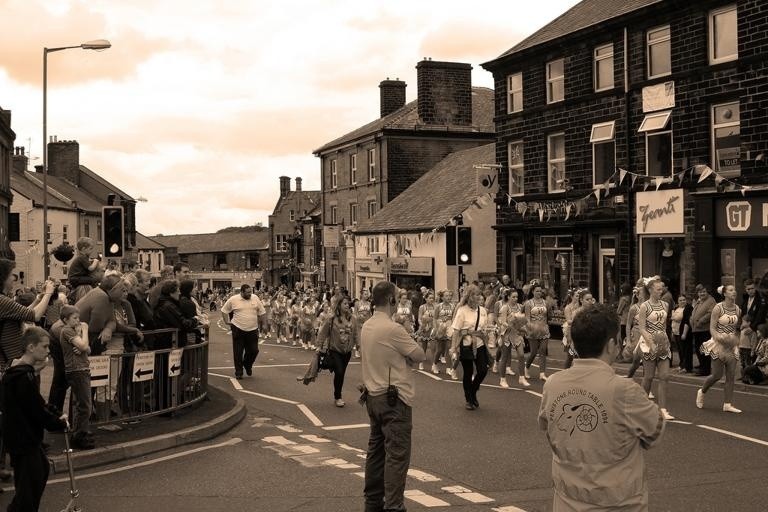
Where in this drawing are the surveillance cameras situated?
[556,178,571,189]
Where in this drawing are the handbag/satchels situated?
[317,352,331,369]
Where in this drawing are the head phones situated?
[389,286,396,306]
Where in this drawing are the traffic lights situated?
[457,227,472,266]
[102,206,124,258]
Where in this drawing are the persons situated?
[0,326,71,512]
[198,281,376,407]
[537,303,666,512]
[615,275,767,420]
[360,280,427,512]
[397,271,596,410]
[1,238,210,447]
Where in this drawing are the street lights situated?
[41,36,112,280]
[108,193,147,205]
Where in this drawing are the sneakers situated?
[695,388,704,409]
[661,408,674,419]
[245,364,252,375]
[184,374,204,394]
[419,354,548,388]
[674,366,704,376]
[465,402,474,410]
[72,441,93,450]
[355,351,361,357]
[51,394,191,437]
[472,398,479,407]
[648,392,655,399]
[236,375,243,379]
[335,399,345,407]
[258,325,317,350]
[0,469,13,484]
[724,402,742,412]
